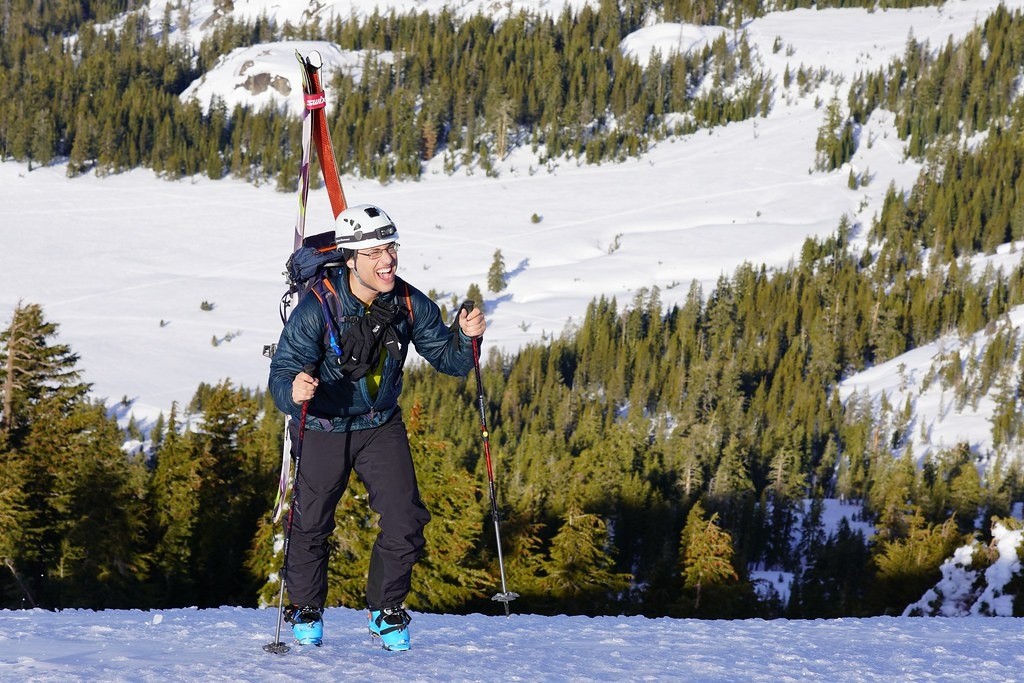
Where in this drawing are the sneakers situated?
[366,604,411,653]
[289,604,324,646]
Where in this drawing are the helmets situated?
[334,204,399,252]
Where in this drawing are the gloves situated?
[340,299,395,367]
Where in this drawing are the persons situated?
[268,203,486,651]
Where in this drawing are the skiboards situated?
[292,47,350,306]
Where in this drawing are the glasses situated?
[357,242,401,259]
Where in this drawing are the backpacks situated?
[285,232,345,285]
